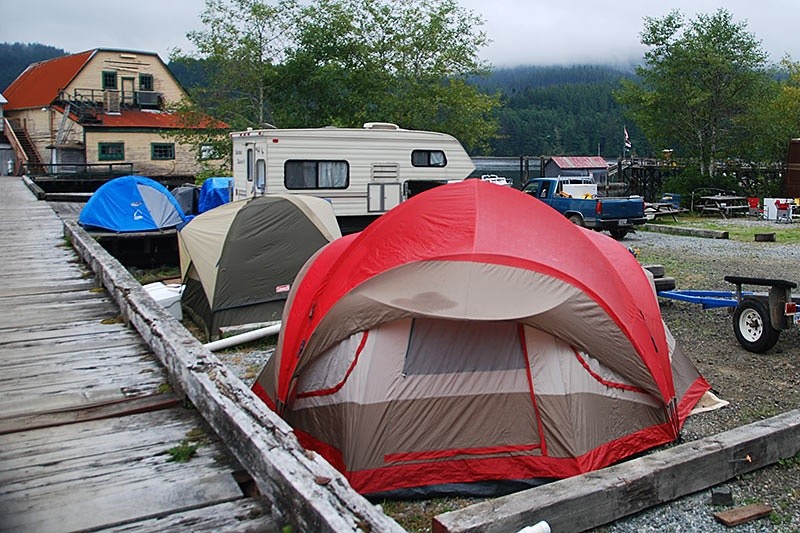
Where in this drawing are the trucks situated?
[229,122,476,234]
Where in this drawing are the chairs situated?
[747,197,770,221]
[775,200,792,225]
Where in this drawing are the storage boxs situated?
[563,184,597,199]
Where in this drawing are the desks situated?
[647,203,678,223]
[700,196,746,220]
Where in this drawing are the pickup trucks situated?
[520,177,648,240]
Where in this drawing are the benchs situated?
[645,210,680,215]
[719,206,749,210]
[695,204,715,207]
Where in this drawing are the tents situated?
[177,194,342,344]
[255,179,711,502]
[80,175,186,232]
